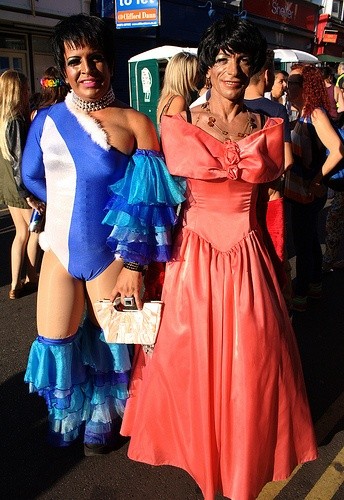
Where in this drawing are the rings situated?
[123,297,134,307]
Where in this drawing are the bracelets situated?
[122,261,145,272]
[309,183,320,186]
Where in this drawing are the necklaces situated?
[72,85,115,111]
[200,102,254,137]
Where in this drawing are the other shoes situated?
[21,282,32,288]
[9,290,16,299]
[291,295,308,311]
[308,283,323,299]
[84,440,104,456]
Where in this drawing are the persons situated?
[20,14,161,458]
[158,52,344,313]
[0,65,71,298]
[120,24,320,500]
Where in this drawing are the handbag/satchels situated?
[94,296,163,345]
